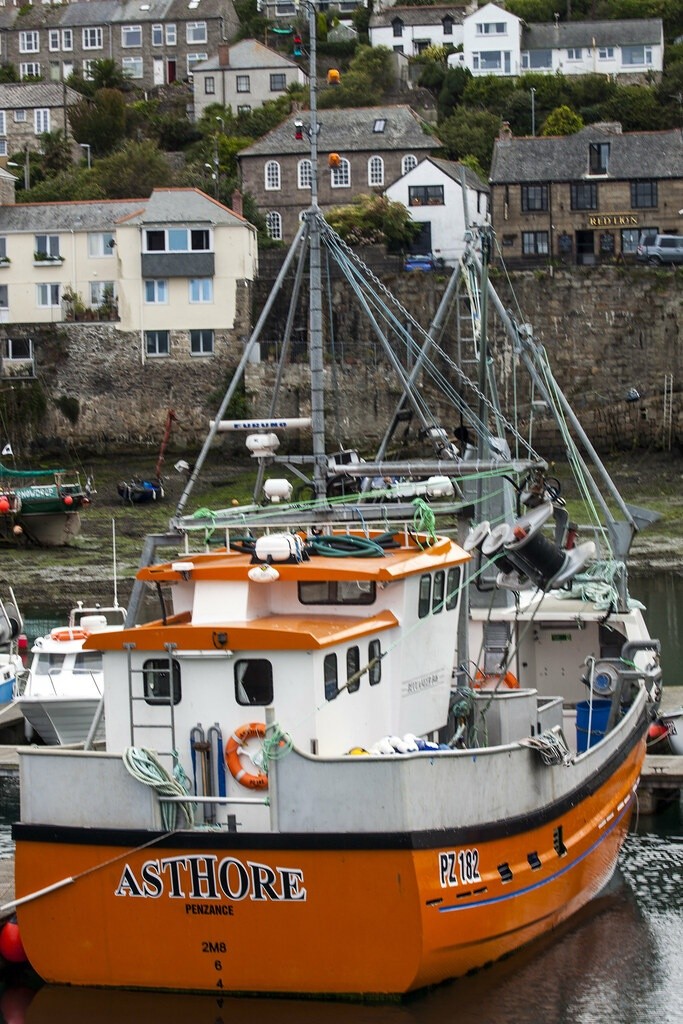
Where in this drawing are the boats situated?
[325,447,367,492]
[116,408,178,501]
[12,597,129,744]
[9,0,660,1001]
[1,590,31,744]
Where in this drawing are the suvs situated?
[638,233,683,267]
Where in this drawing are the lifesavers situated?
[472,667,521,689]
[224,722,284,792]
[51,629,92,641]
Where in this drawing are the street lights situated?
[7,163,28,189]
[216,117,224,131]
[79,144,89,169]
[531,86,536,130]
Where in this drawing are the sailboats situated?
[0,345,96,547]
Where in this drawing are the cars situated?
[403,251,432,273]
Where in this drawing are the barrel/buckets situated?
[576,698,609,755]
